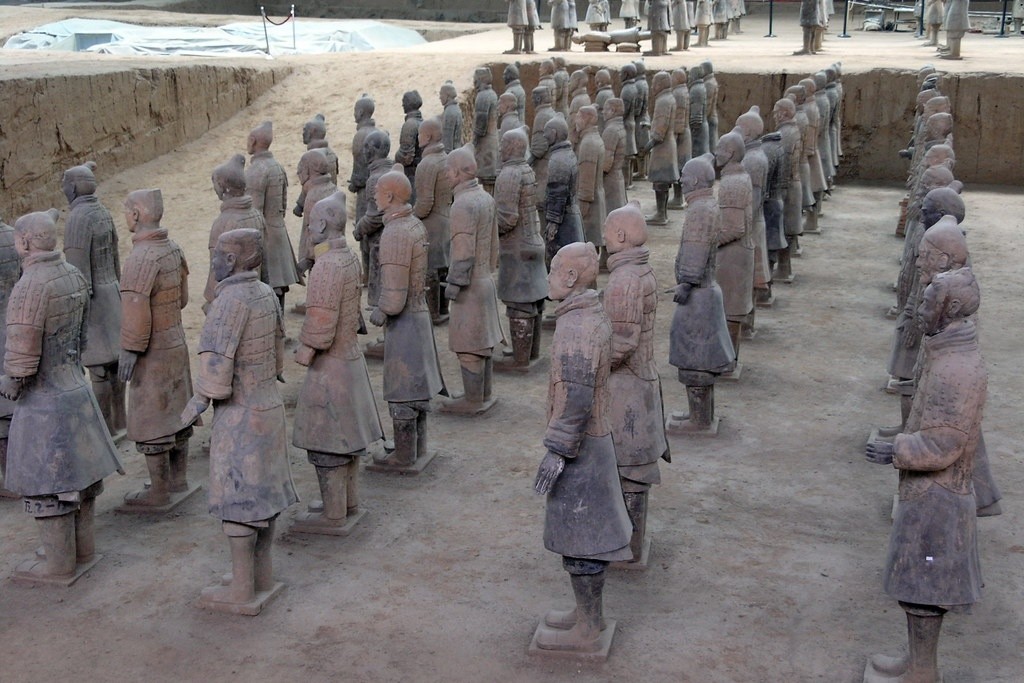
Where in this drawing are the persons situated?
[600,199,671,564]
[181,229,302,603]
[0,209,124,578]
[367,162,449,467]
[441,141,509,408]
[865,68,1003,683]
[714,61,842,343]
[118,188,204,504]
[294,57,718,360]
[666,151,735,431]
[0,219,24,498]
[502,0,640,54]
[793,0,834,55]
[643,0,747,56]
[60,161,127,434]
[531,240,633,654]
[292,191,388,527]
[912,0,970,57]
[203,121,307,391]
[1011,0,1024,35]
